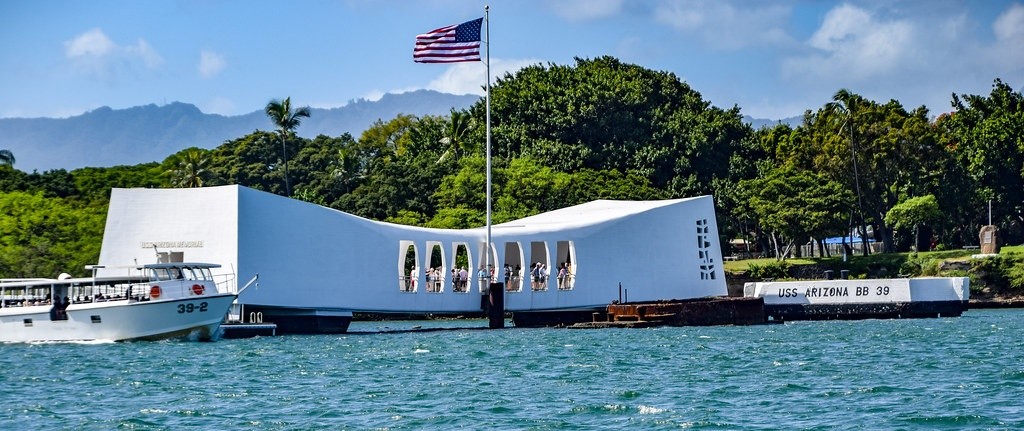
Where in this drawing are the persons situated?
[408,261,571,292]
[125,286,133,299]
[177,270,184,279]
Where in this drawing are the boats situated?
[0,251,263,345]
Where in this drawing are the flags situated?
[414,16,482,62]
